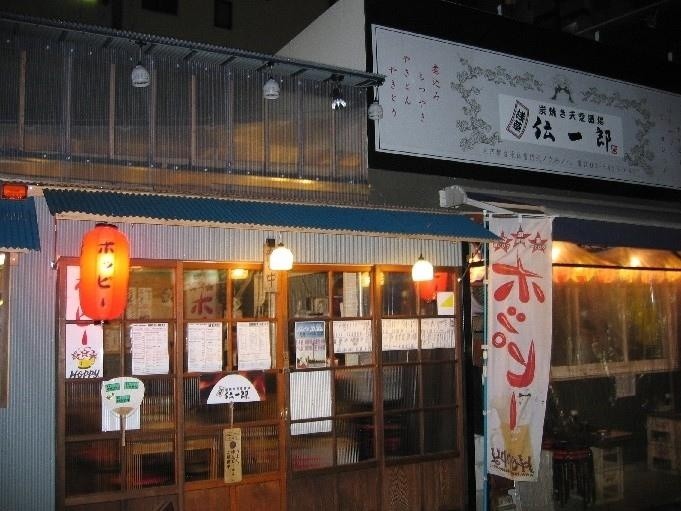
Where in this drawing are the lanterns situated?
[77,223,132,323]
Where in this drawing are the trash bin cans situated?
[553,449,596,511]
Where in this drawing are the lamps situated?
[132,41,151,88]
[263,62,281,100]
[368,82,383,121]
[412,239,433,281]
[269,231,293,271]
